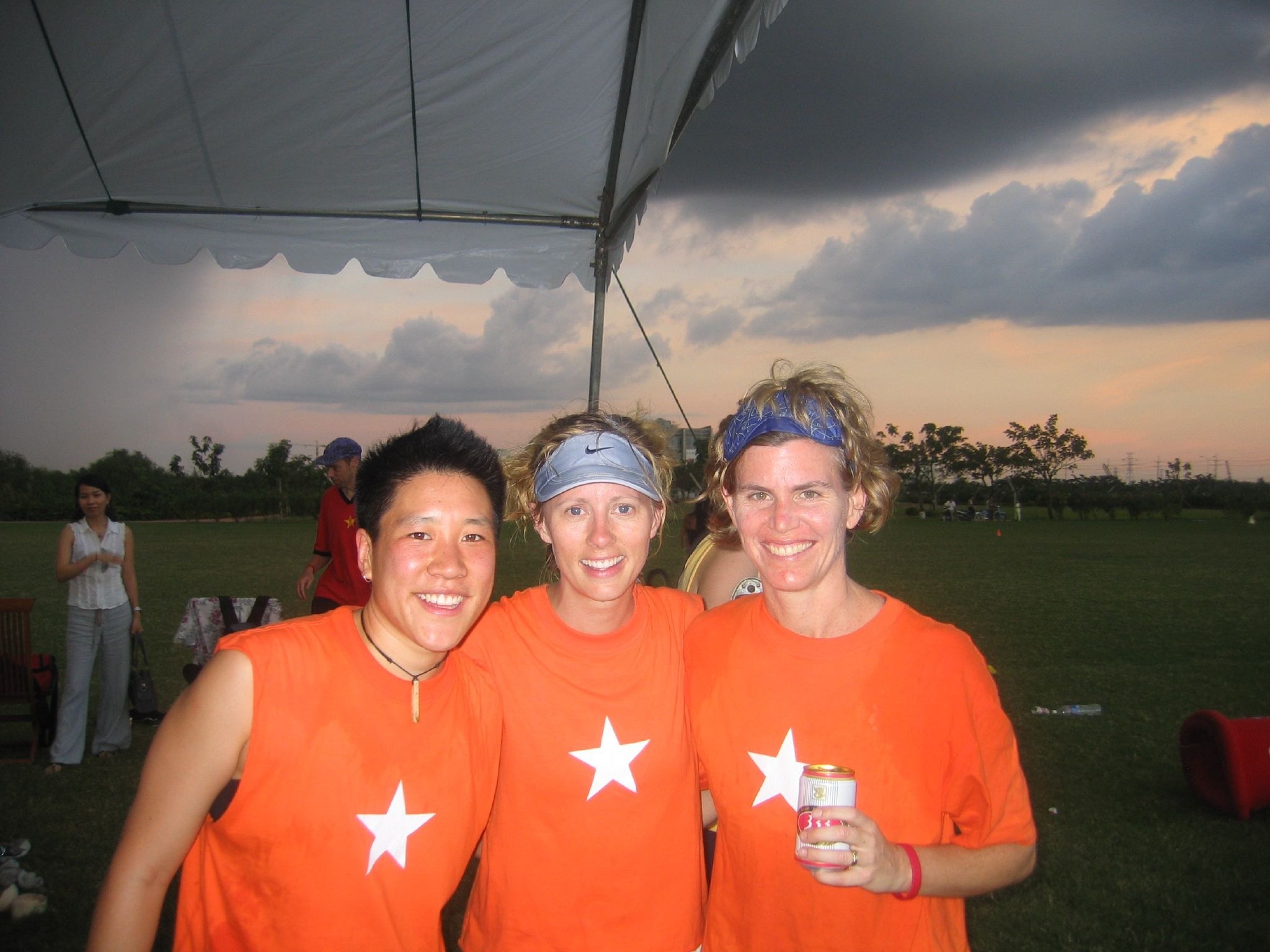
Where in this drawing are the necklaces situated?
[97,533,102,537]
[361,609,450,723]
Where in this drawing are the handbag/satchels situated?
[127,631,159,715]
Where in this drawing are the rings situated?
[850,850,858,864]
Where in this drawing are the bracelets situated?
[92,550,97,562]
[132,606,142,613]
[306,565,314,574]
[892,843,921,901]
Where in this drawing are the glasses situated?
[99,546,108,573]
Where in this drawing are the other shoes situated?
[96,749,117,765]
[43,762,64,777]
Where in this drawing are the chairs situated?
[0,596,59,764]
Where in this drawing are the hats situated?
[314,437,362,466]
[532,432,663,505]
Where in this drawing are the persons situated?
[47,474,144,774]
[297,438,372,616]
[686,359,1036,952]
[456,412,718,952]
[85,416,505,952]
[682,487,759,884]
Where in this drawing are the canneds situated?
[794,764,858,871]
